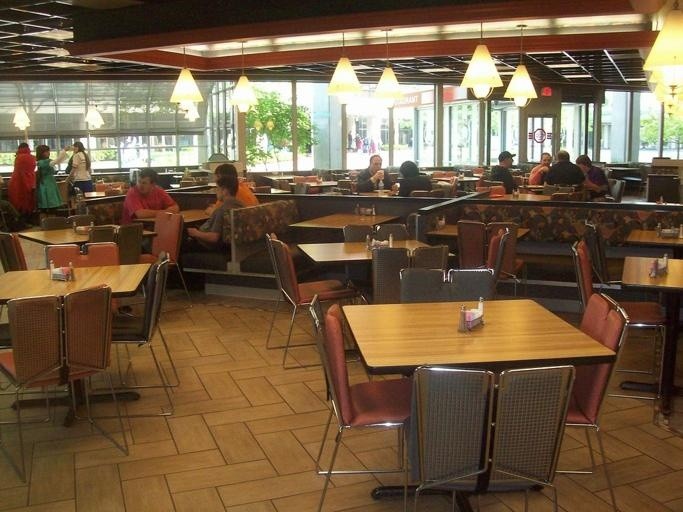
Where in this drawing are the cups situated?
[512,189,519,200]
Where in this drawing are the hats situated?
[497,150,517,160]
[70,142,84,148]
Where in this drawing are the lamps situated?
[10,81,30,132]
[83,82,105,129]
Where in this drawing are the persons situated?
[346,130,376,153]
[201,164,260,217]
[356,155,394,193]
[32,144,72,217]
[385,159,433,197]
[487,150,517,194]
[119,167,180,254]
[3,143,37,223]
[526,152,552,195]
[574,156,609,202]
[168,175,249,290]
[542,150,586,192]
[63,140,93,197]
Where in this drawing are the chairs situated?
[398,362,578,512]
[547,288,632,510]
[0,283,138,485]
[301,292,417,511]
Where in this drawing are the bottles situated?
[372,207,375,215]
[378,180,384,196]
[67,262,75,281]
[72,222,76,233]
[649,254,668,278]
[365,235,370,250]
[49,260,54,279]
[349,180,359,194]
[389,233,393,248]
[477,296,484,323]
[678,224,682,239]
[457,305,468,333]
[656,222,661,237]
